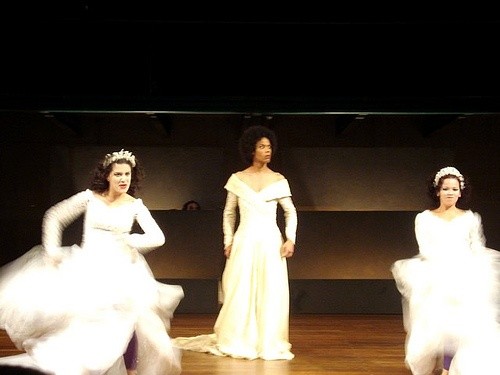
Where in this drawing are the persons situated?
[0,149,184,375]
[171,124,297,361]
[390,166,500,375]
[182,200,200,210]
